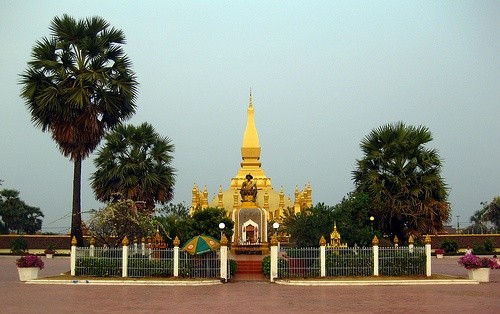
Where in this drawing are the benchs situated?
[457,248,473,253]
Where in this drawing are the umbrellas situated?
[181,234,221,256]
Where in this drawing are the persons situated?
[239,173,257,202]
[464,245,473,256]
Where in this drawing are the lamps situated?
[273,223,279,235]
[219,223,225,235]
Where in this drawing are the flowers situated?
[44,250,55,254]
[16,255,45,270]
[458,253,500,270]
[435,249,445,254]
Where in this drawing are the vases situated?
[18,267,38,281]
[468,268,491,282]
[436,254,444,258]
[46,254,54,258]
